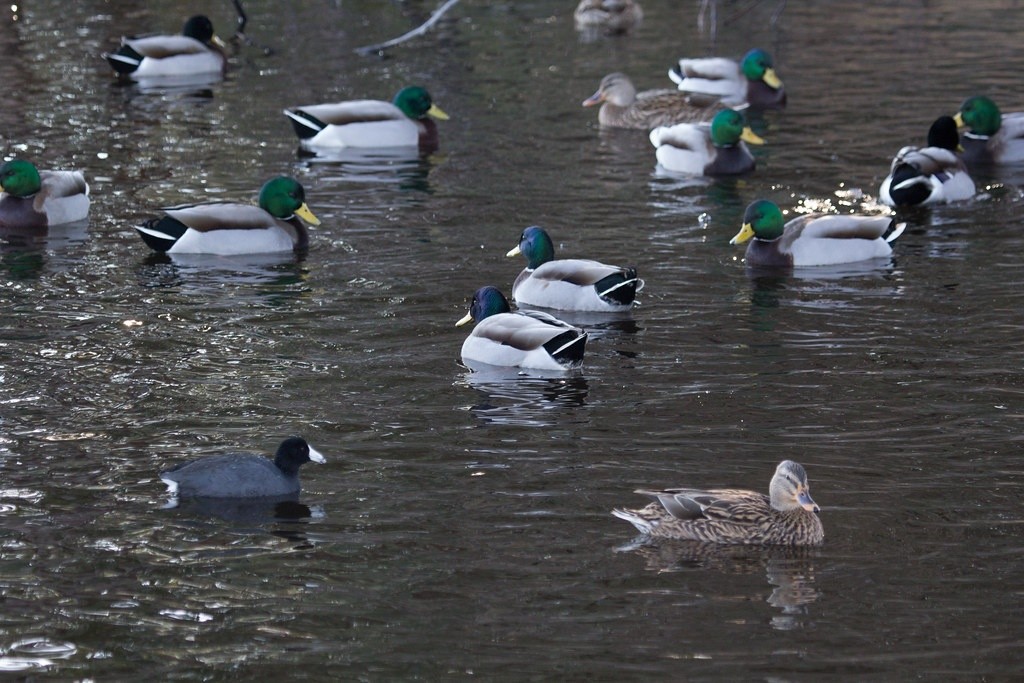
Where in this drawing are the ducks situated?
[611,460,824,546]
[582,72,727,131]
[573,0,644,34]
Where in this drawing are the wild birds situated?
[649,107,766,175]
[0,160,90,228]
[106,15,227,75]
[668,47,787,111]
[879,116,976,206]
[159,438,327,497]
[952,96,1024,164]
[282,84,451,147]
[455,286,589,370]
[729,199,906,268]
[128,176,321,255]
[506,227,644,312]
